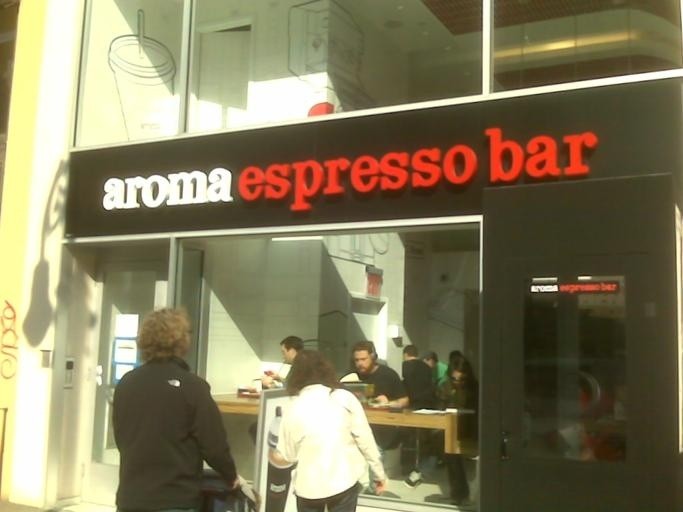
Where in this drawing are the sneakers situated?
[403,471,422,487]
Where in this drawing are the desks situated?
[206,390,458,494]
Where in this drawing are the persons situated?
[267,349,387,512]
[112,307,257,512]
[339,338,479,508]
[263,337,304,389]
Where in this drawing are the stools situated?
[383,436,403,480]
